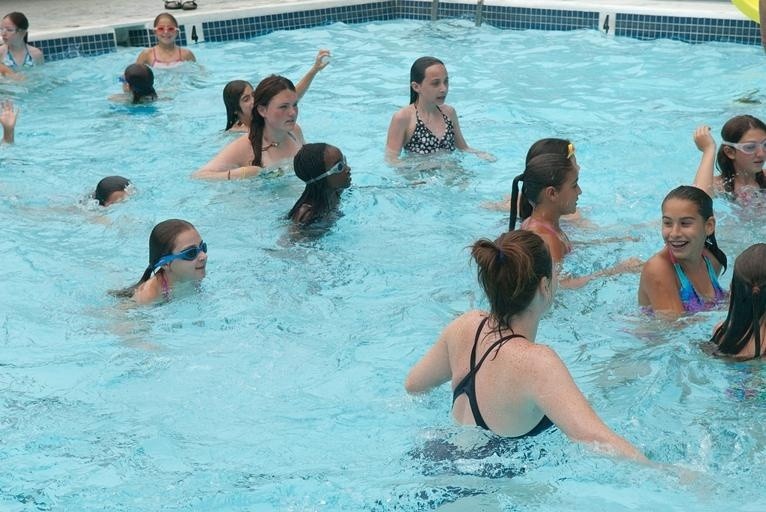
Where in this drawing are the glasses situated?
[118,76,128,85]
[328,155,348,177]
[172,239,208,262]
[564,143,578,159]
[155,26,176,33]
[734,139,766,154]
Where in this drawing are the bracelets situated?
[227,169,231,181]
[240,166,245,179]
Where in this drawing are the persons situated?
[190,75,306,184]
[0,12,44,82]
[383,55,498,193]
[0,98,19,145]
[402,229,706,511]
[96,217,208,354]
[135,12,206,74]
[506,152,646,292]
[689,113,766,233]
[637,185,732,346]
[267,142,354,261]
[14,175,136,230]
[483,138,665,234]
[221,48,332,134]
[106,62,173,105]
[709,243,766,405]
